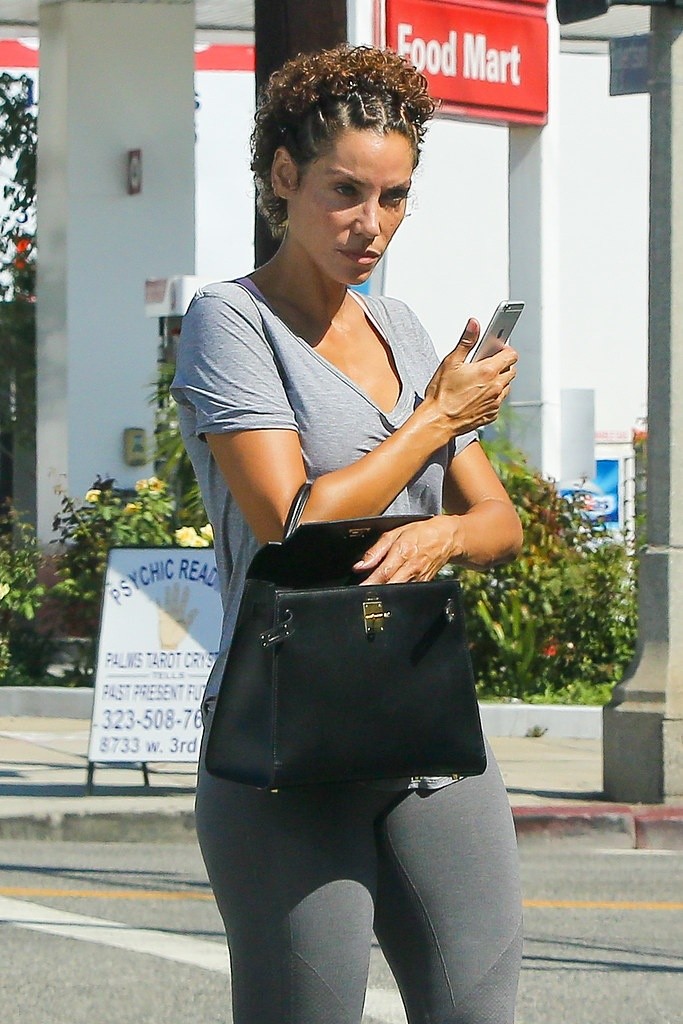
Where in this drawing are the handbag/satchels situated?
[204,481,489,794]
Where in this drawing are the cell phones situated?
[470,300,524,363]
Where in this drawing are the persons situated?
[170,47,564,1024]
